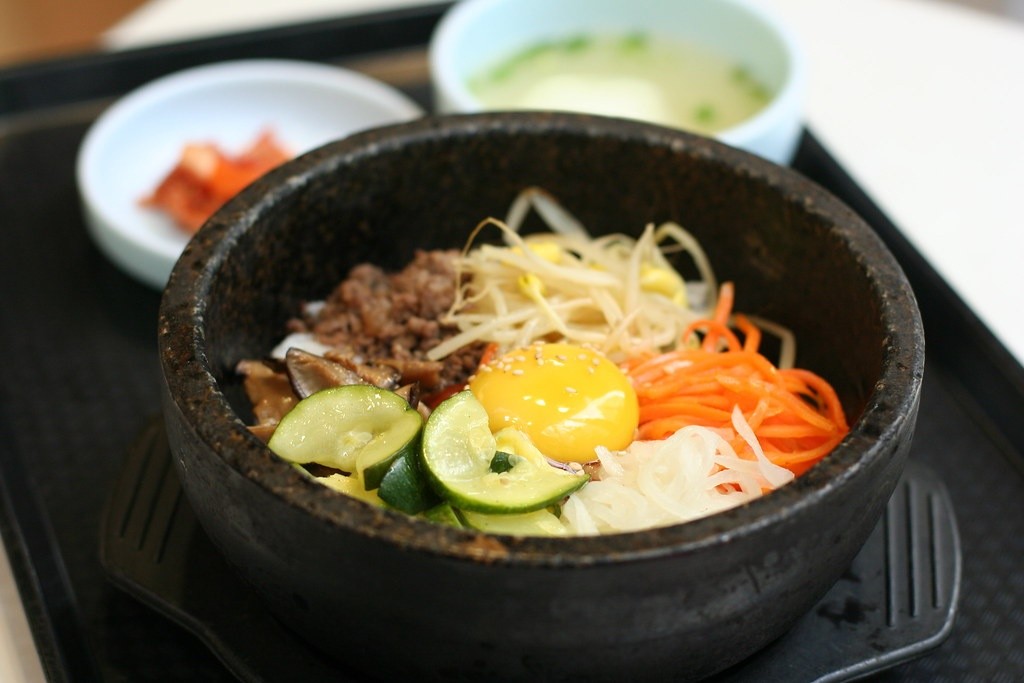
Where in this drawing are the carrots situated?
[617,281,852,482]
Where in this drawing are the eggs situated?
[465,339,638,465]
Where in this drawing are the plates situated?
[74,57,429,291]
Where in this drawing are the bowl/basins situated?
[157,109,925,683]
[427,0,807,168]
